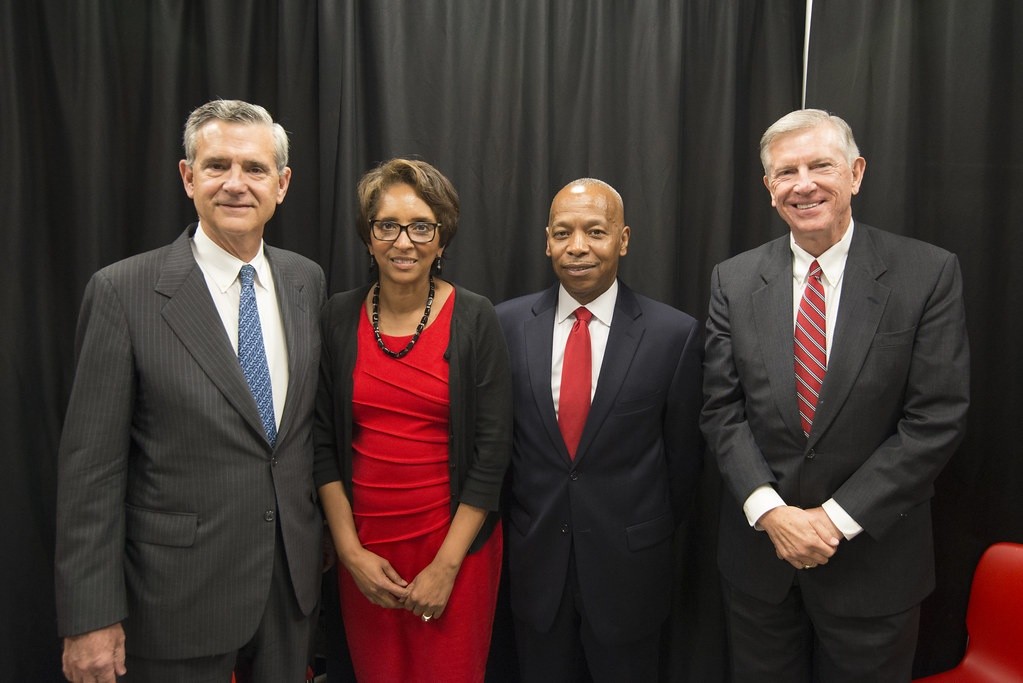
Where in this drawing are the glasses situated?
[367,217,441,244]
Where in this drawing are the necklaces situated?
[372,276,435,359]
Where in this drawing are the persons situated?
[56,97,329,683]
[311,158,514,683]
[697,109,972,683]
[487,177,703,683]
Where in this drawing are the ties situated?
[795,260,826,439]
[558,306,594,461]
[236,263,276,451]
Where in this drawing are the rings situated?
[805,562,814,569]
[422,614,433,621]
